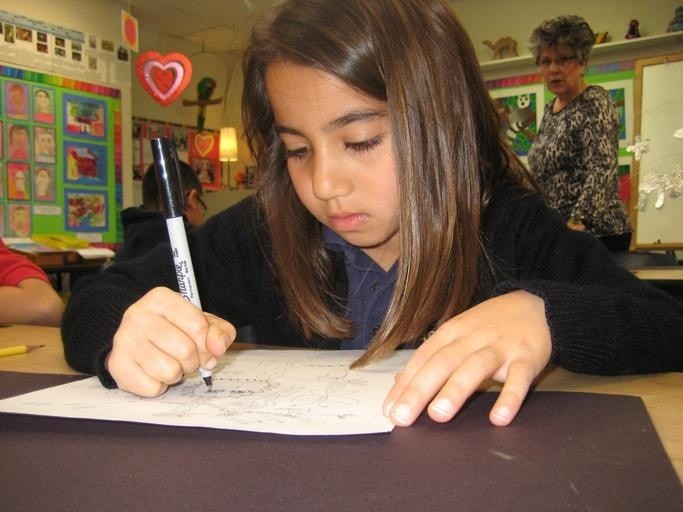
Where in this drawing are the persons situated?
[4,78,57,237]
[0,236,64,326]
[111,155,208,261]
[59,2,682,428]
[523,12,633,255]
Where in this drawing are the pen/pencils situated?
[151,136,212,393]
[0,344,45,357]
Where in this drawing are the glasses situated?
[184,190,207,217]
[536,54,577,69]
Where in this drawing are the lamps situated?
[219,126,238,190]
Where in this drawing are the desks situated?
[0,322,683,510]
[38,260,107,305]
